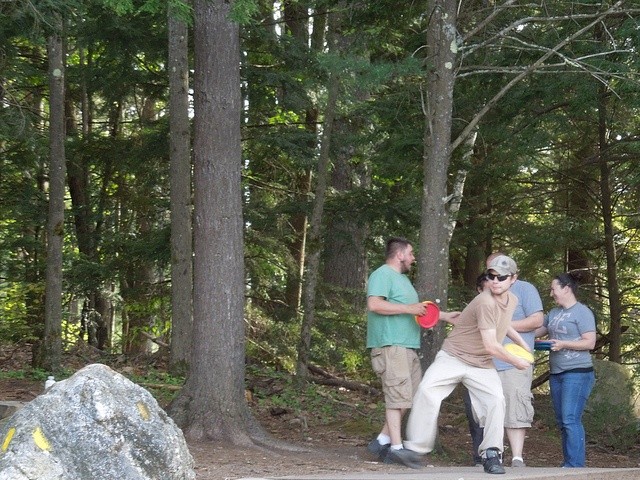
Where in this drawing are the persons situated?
[463,273,504,467]
[366,238,463,468]
[486,253,544,468]
[534,273,596,467]
[403,256,531,474]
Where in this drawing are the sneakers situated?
[393,449,427,469]
[367,438,392,461]
[481,447,504,474]
[510,459,528,467]
[382,446,399,465]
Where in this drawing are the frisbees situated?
[503,343,534,363]
[417,303,440,329]
[534,340,555,345]
[415,301,434,324]
[534,345,553,350]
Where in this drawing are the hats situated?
[485,255,517,277]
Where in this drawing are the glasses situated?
[488,273,513,280]
